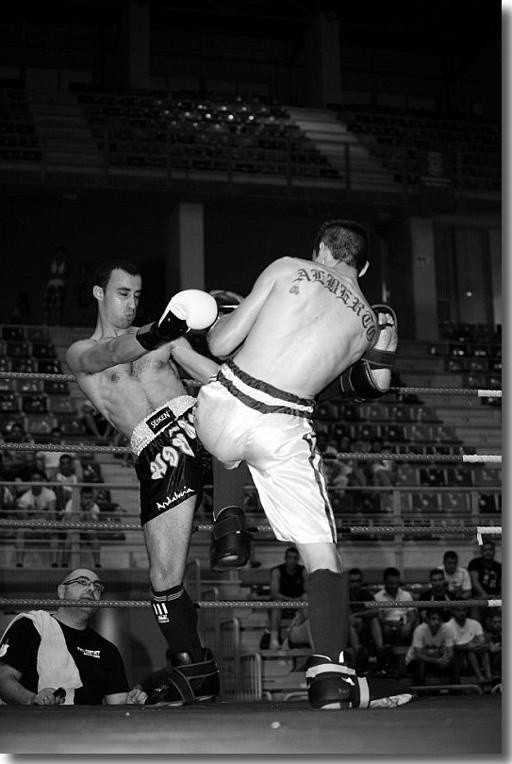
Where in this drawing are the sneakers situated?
[304,649,416,713]
[204,502,256,573]
[127,642,220,711]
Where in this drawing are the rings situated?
[44,697,47,701]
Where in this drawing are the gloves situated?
[135,287,219,351]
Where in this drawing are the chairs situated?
[0,325,127,543]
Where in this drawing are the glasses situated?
[63,576,106,588]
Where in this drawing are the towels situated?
[0,609,83,705]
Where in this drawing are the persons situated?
[1,568,148,707]
[65,257,259,707]
[318,433,395,507]
[347,540,499,682]
[270,546,308,651]
[0,432,148,566]
[191,215,415,710]
